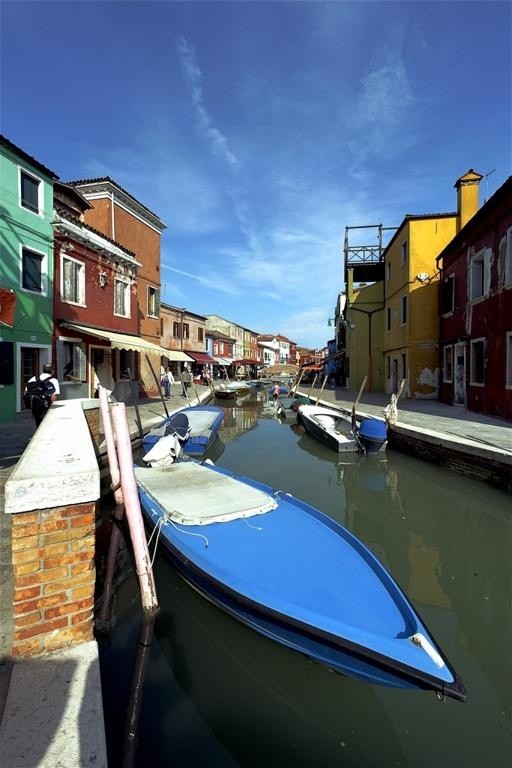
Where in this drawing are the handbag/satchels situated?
[160,379,164,386]
[187,382,192,387]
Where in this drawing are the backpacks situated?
[24,376,53,410]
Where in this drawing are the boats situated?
[130,455,472,706]
[298,403,389,456]
[142,404,227,459]
[278,397,309,418]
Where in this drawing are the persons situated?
[160,365,177,399]
[201,366,227,386]
[179,367,190,397]
[24,362,61,427]
[273,383,280,407]
